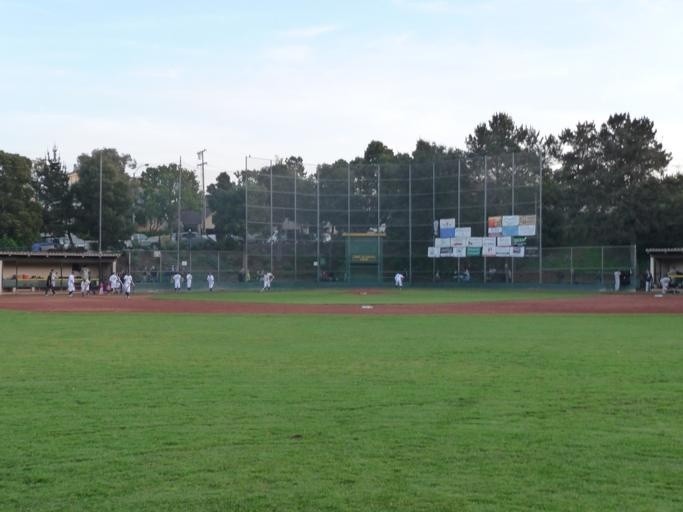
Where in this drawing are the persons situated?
[206,271,215,292]
[612,265,671,296]
[185,271,192,291]
[260,271,274,293]
[393,271,404,287]
[42,265,136,300]
[140,262,158,284]
[171,271,180,293]
[238,263,261,282]
